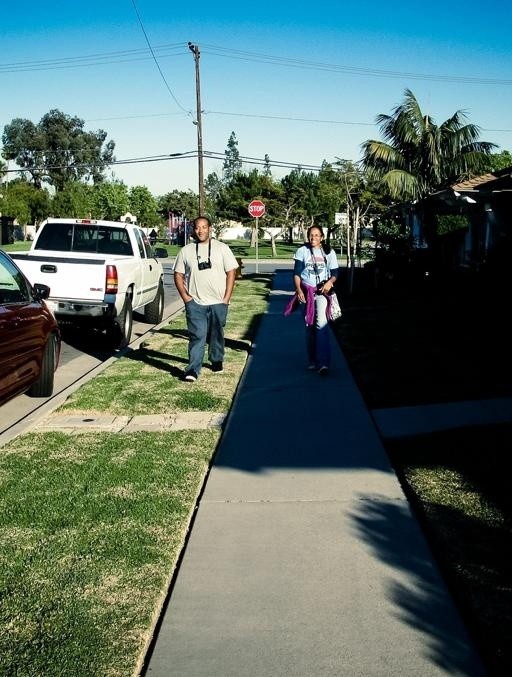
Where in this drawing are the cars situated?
[0,251,62,406]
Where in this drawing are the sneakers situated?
[185,369,197,381]
[309,364,328,375]
[211,362,223,373]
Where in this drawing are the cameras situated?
[198,262,212,270]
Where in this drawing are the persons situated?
[170,215,239,382]
[149,228,158,245]
[291,225,340,374]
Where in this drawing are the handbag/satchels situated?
[317,280,334,295]
[314,293,342,329]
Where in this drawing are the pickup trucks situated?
[1,218,165,350]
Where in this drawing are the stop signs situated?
[248,200,265,218]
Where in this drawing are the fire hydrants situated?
[235,258,244,278]
